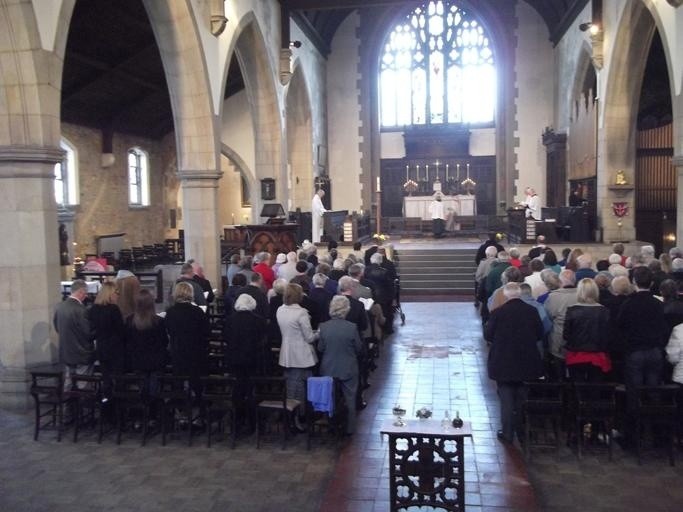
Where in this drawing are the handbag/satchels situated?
[179,417,205,435]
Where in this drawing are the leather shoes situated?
[359,400,367,409]
[498,430,507,440]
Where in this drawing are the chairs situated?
[31,298,348,450]
[86,244,178,273]
[517,359,681,466]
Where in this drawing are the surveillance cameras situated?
[289,41,301,48]
[579,22,591,30]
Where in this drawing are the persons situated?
[312,188,327,243]
[520,189,542,221]
[164,239,400,437]
[475,231,683,442]
[427,195,447,237]
[523,187,532,219]
[54,269,169,432]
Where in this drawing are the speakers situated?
[261,178,275,199]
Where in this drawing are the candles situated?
[406,164,469,182]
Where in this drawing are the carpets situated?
[400,237,482,243]
